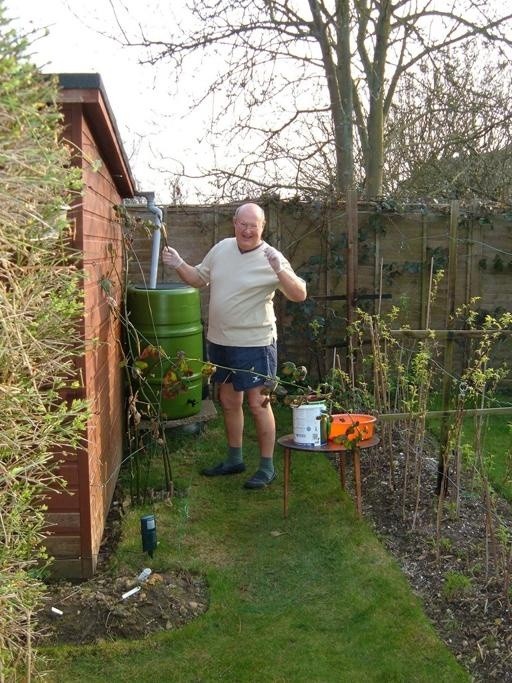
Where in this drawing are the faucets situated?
[187,399,198,407]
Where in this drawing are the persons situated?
[160,200,309,491]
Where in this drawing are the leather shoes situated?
[202,461,246,476]
[244,466,278,488]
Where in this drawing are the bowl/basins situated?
[329,410,377,440]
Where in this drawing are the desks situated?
[277,433,381,519]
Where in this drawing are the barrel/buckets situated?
[125,279,205,421]
[292,404,330,447]
